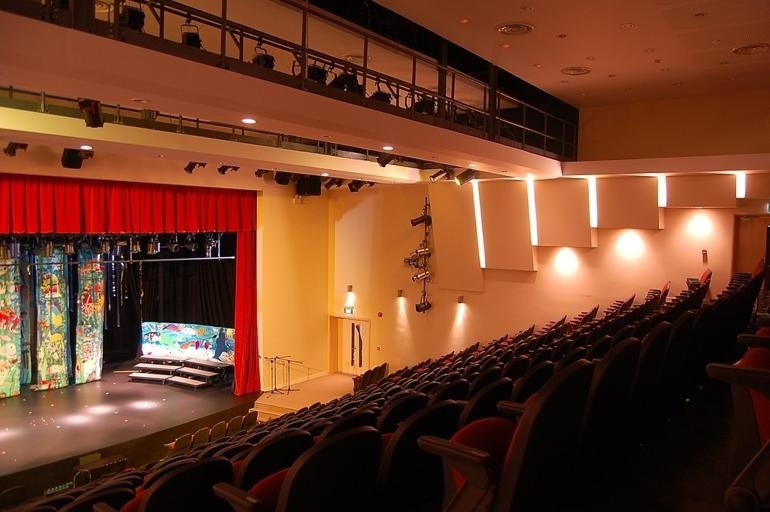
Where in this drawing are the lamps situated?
[0,234,219,257]
[347,179,364,193]
[2,138,24,159]
[411,94,436,116]
[370,83,393,104]
[270,166,291,188]
[373,147,393,169]
[456,110,469,124]
[428,164,450,185]
[253,166,267,179]
[183,159,207,178]
[78,98,107,131]
[329,64,360,93]
[118,5,147,31]
[247,40,279,70]
[453,167,475,189]
[324,176,344,192]
[407,197,432,313]
[304,58,328,85]
[217,163,240,178]
[179,21,204,48]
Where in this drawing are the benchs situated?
[130,352,236,390]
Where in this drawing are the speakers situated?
[295,175,321,196]
[60,149,82,169]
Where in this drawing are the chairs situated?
[9,258,770,512]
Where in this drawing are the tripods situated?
[263,358,300,398]
